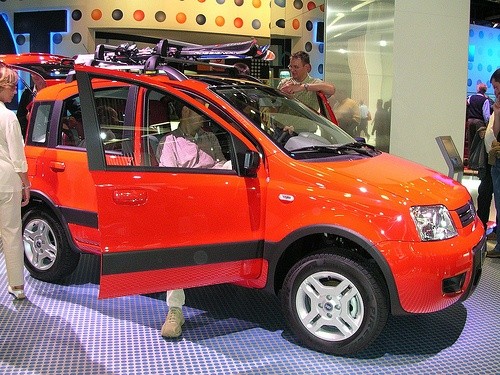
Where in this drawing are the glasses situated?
[287,63,304,71]
[8,83,17,92]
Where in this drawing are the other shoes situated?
[7,285,25,299]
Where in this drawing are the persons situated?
[155,97,233,338]
[234,63,250,81]
[0,68,32,299]
[78,105,119,150]
[463,68,500,257]
[269,51,336,141]
[236,94,295,140]
[329,85,392,154]
[17,85,82,144]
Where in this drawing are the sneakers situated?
[486,230,500,241]
[161,307,185,340]
[487,244,500,258]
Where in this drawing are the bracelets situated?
[22,182,32,189]
[304,84,308,91]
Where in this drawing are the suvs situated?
[0,37,488,355]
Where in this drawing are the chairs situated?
[150,100,171,167]
[63,115,85,145]
